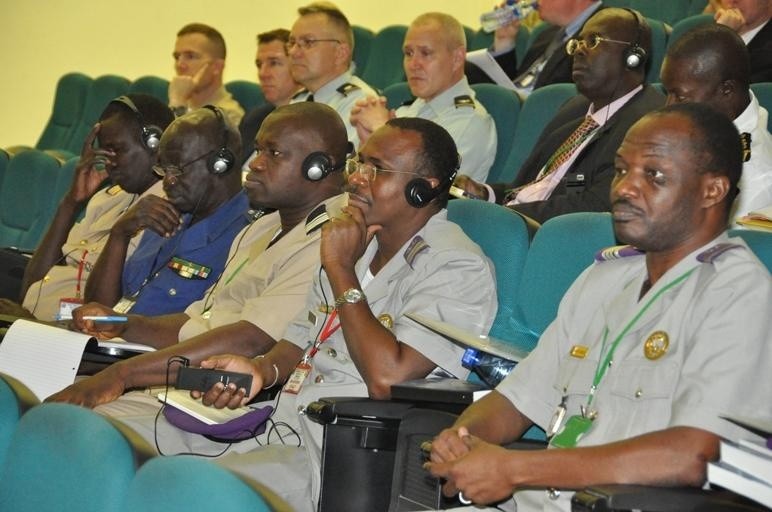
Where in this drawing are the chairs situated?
[726,227,772,271]
[458,20,579,186]
[747,81,772,131]
[444,198,541,339]
[120,452,297,512]
[350,23,418,108]
[225,79,267,111]
[500,210,616,356]
[602,1,717,100]
[0,72,170,253]
[1,402,157,510]
[0,371,42,451]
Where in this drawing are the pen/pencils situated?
[51,315,128,323]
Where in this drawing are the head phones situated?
[621,7,649,73]
[302,140,356,182]
[110,94,163,154]
[403,154,463,208]
[202,104,237,177]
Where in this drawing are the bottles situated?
[460,348,516,386]
[478,1,539,36]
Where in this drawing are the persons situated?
[0,2,772,511]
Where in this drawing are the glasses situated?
[286,38,341,54]
[151,150,214,178]
[345,157,419,182]
[565,33,630,56]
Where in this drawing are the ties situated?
[511,115,600,194]
